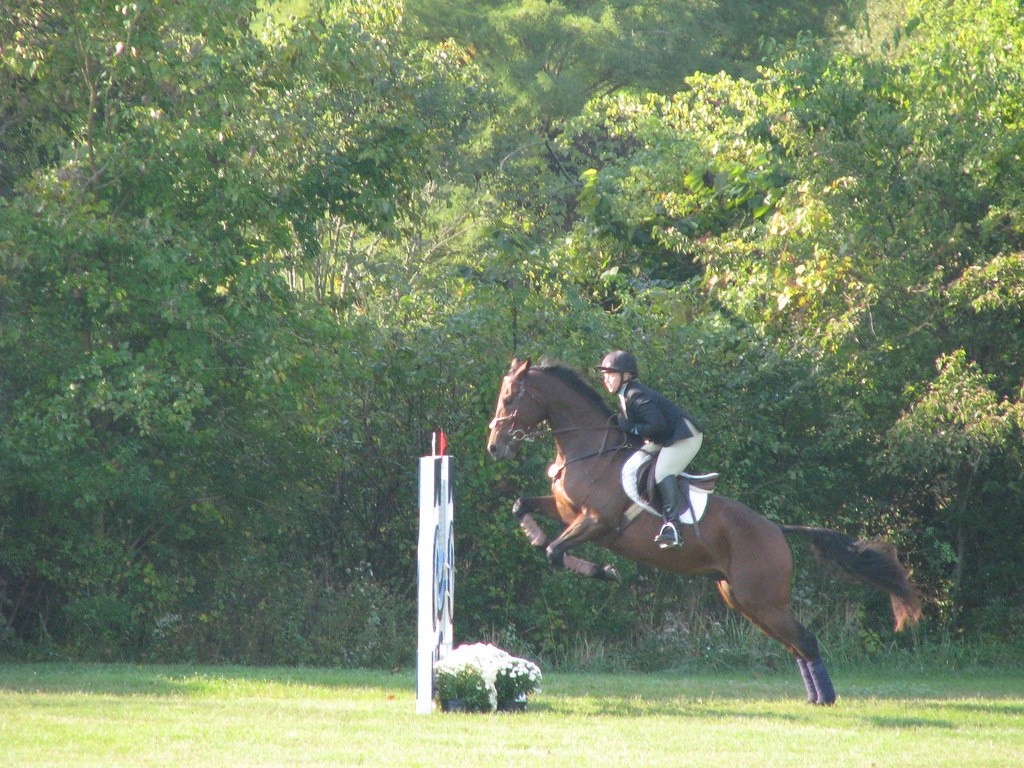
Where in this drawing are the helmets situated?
[595,349,638,377]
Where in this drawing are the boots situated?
[654,475,685,543]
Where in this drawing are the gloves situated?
[618,415,634,433]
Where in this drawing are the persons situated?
[594,348,704,551]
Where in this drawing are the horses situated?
[485,356,921,707]
